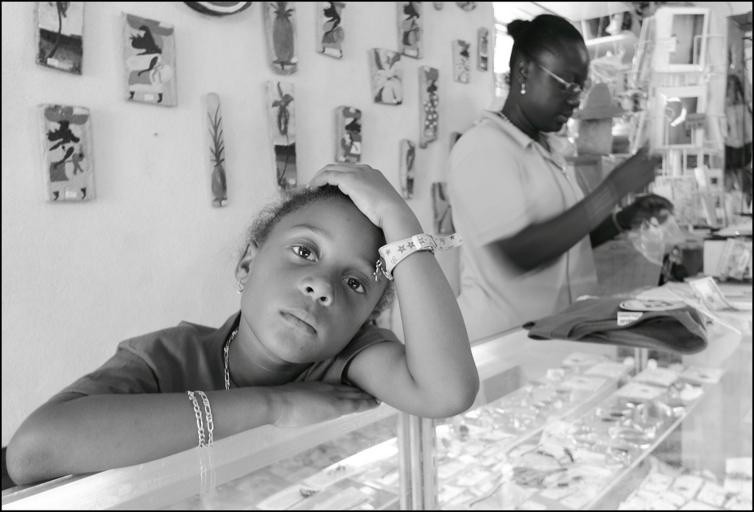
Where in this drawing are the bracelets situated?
[185,388,215,448]
[198,449,216,498]
[611,211,625,232]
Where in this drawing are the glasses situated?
[540,65,582,95]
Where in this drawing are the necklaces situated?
[222,327,239,390]
[497,110,511,123]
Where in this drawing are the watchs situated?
[377,231,465,282]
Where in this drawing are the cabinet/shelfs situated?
[1,280,752,510]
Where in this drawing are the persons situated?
[445,13,676,340]
[4,158,480,487]
